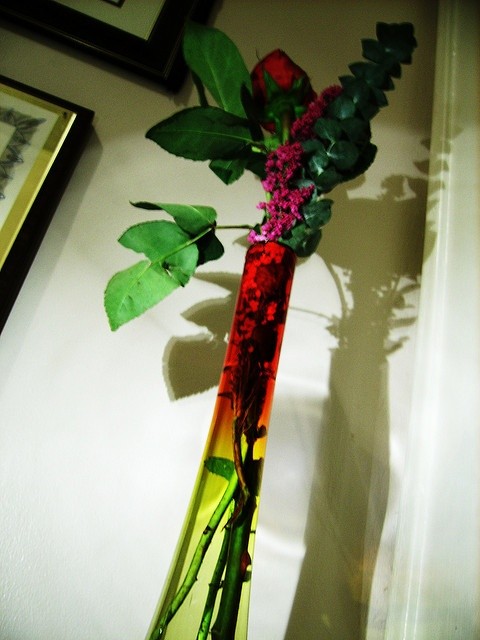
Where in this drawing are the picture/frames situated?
[0,0,221,97]
[0,74,96,335]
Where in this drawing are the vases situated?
[145,240,297,640]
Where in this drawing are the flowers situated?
[104,21,418,640]
